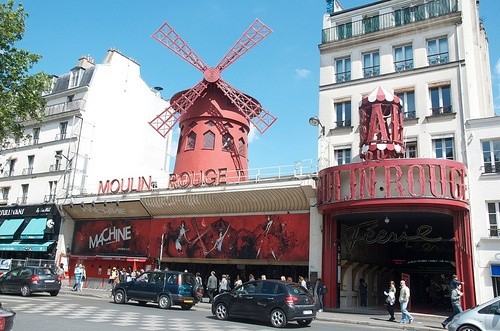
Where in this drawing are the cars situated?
[211,279,317,329]
[0,265,62,298]
[442,293,500,330]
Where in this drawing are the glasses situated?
[390,283,394,284]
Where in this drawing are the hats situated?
[113,267,116,269]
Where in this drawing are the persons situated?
[0,263,465,328]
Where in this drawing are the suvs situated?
[111,270,203,309]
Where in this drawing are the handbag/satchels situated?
[114,277,120,284]
[81,276,85,282]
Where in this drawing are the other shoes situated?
[400,321,406,324]
[389,317,395,321]
[441,323,446,329]
[318,309,323,313]
[409,318,414,324]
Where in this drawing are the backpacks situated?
[320,285,328,295]
